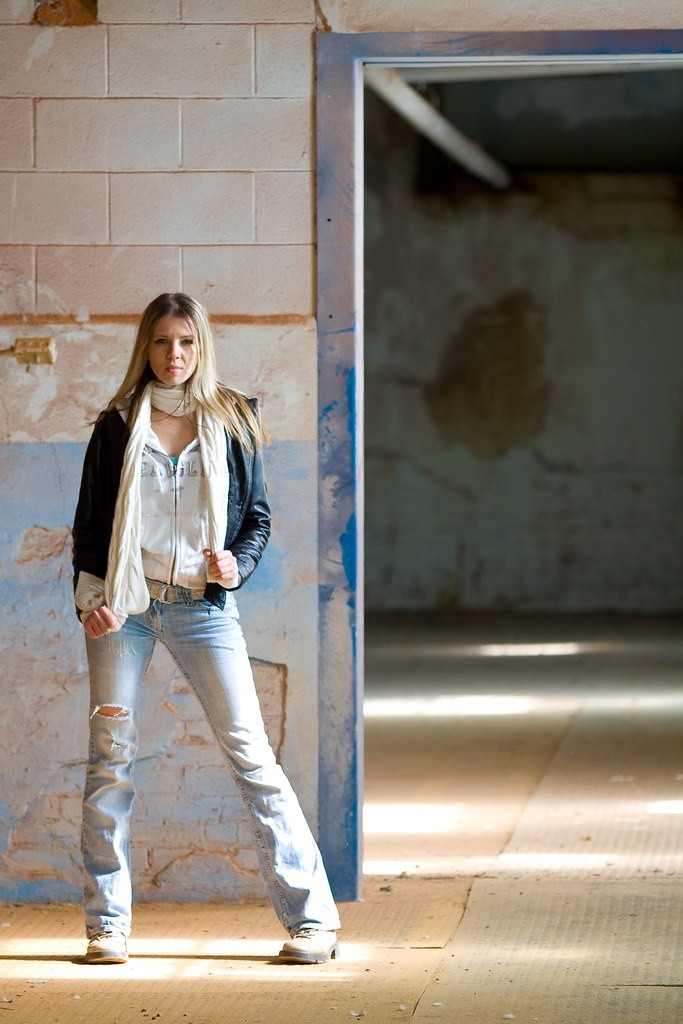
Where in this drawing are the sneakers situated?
[279,928,339,962]
[86,931,128,963]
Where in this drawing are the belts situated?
[146,580,207,604]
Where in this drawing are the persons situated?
[72,292,342,964]
